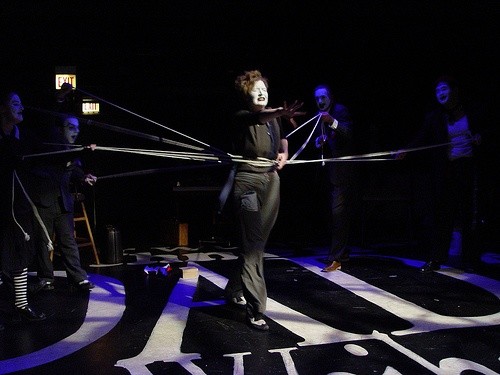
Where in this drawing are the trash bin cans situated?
[102,223,123,264]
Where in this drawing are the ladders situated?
[49,192,101,266]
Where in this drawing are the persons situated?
[391,77,490,273]
[304,84,360,273]
[225,70,308,331]
[0,86,99,321]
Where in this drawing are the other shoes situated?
[233,294,247,305]
[323,261,342,272]
[416,260,441,276]
[248,316,270,331]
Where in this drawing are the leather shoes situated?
[0,323,7,335]
[77,280,95,289]
[11,308,46,321]
[42,284,54,290]
